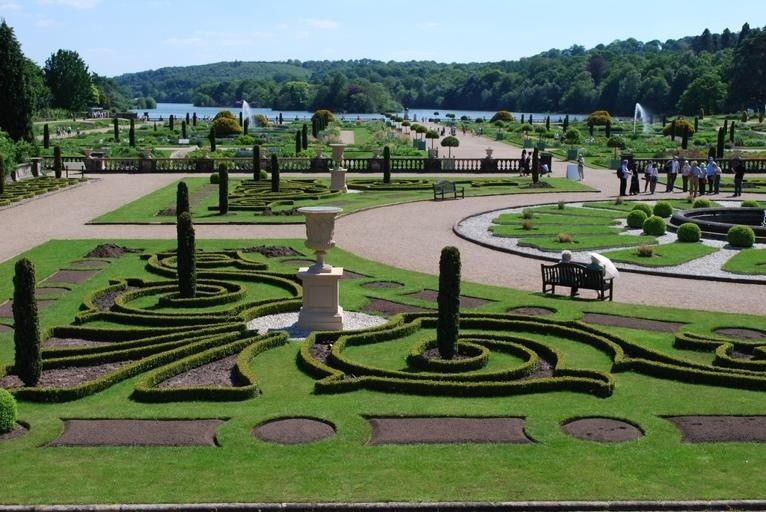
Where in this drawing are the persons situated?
[450,125,456,137]
[558,249,581,296]
[698,162,707,196]
[706,156,715,194]
[619,160,632,196]
[712,162,723,195]
[559,134,567,144]
[462,124,466,134]
[629,163,640,196]
[681,159,691,192]
[664,155,681,192]
[644,160,653,193]
[732,159,746,196]
[584,255,608,299]
[576,154,585,182]
[649,162,659,194]
[519,150,527,176]
[523,152,533,177]
[688,160,703,198]
[476,125,481,138]
[554,131,560,141]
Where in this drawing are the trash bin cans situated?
[91,152,106,171]
[538,151,553,173]
[567,164,579,180]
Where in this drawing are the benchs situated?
[541,261,615,302]
[432,179,465,200]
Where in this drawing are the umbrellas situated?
[586,250,621,281]
